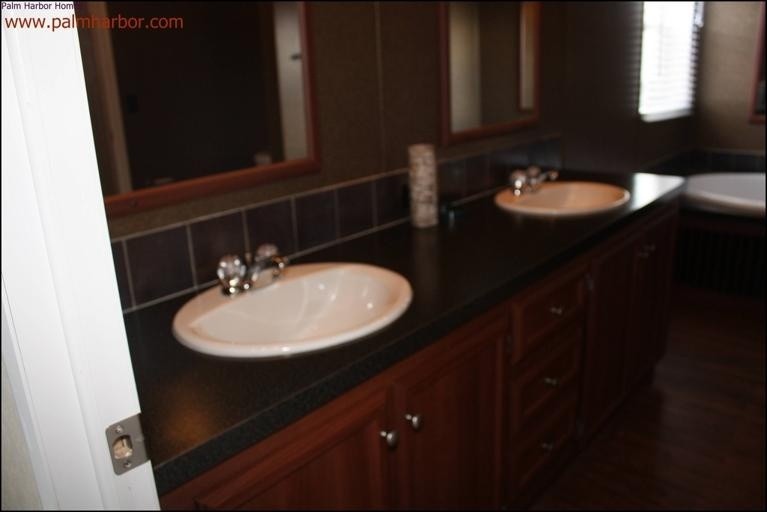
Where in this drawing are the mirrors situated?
[70,1,324,220]
[436,4,544,146]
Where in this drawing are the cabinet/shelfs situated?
[161,192,682,511]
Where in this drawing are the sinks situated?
[493,181,631,217]
[171,262,415,360]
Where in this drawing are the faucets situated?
[525,165,559,193]
[240,251,290,287]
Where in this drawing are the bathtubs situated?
[684,171,766,217]
